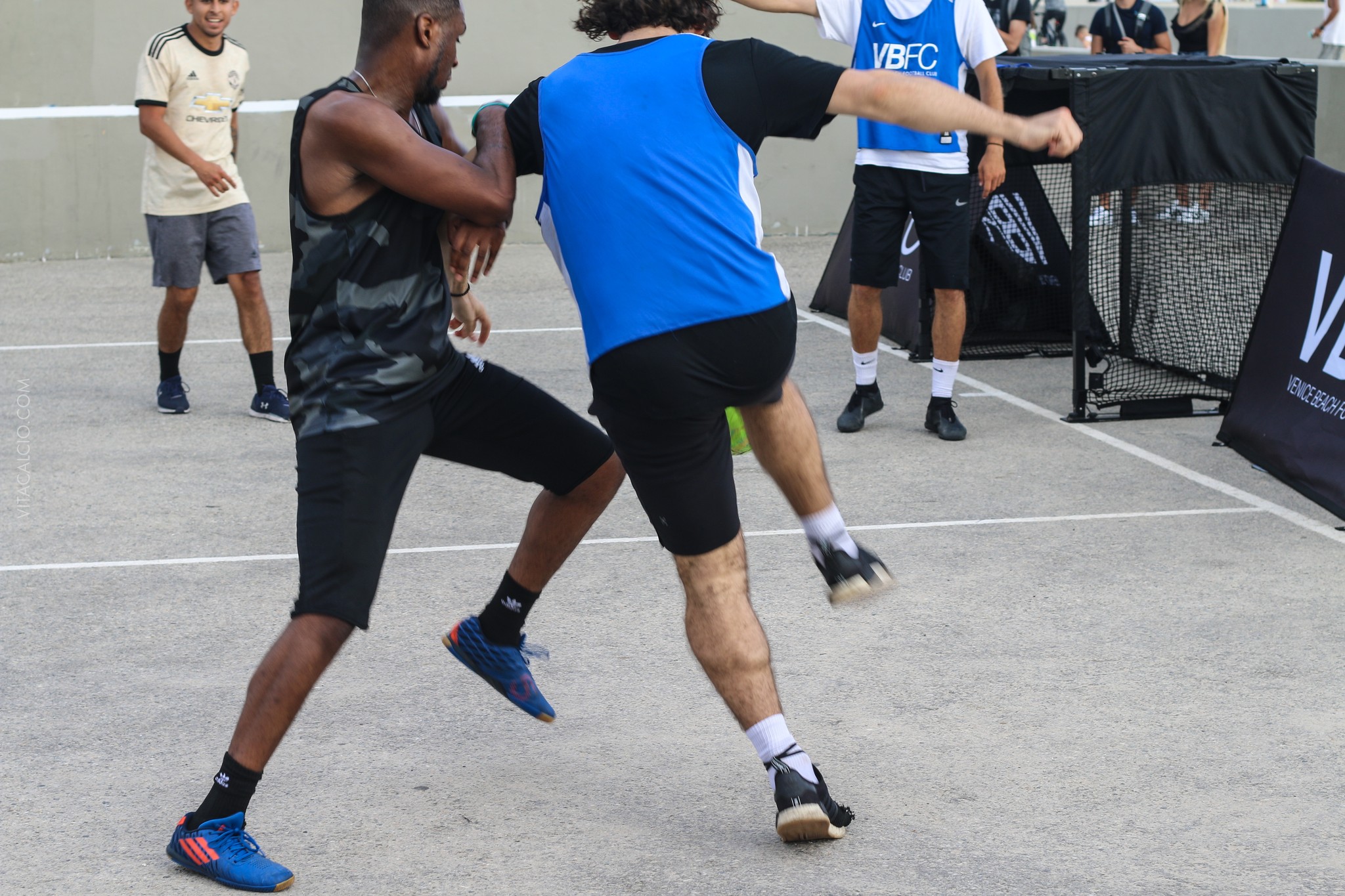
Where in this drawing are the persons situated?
[1089,0,1172,227]
[983,0,1031,56]
[1067,25,1092,48]
[731,0,1009,441]
[166,0,626,893]
[1156,0,1229,224]
[1310,0,1345,60]
[135,0,291,423]
[1031,0,1067,47]
[439,0,1084,844]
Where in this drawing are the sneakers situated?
[837,383,884,434]
[811,543,894,607]
[247,384,292,424]
[155,379,192,414]
[924,397,968,441]
[440,612,559,725]
[770,753,857,843]
[165,810,295,893]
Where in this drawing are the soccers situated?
[725,406,752,456]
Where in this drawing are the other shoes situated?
[1088,205,1113,227]
[1154,198,1211,224]
[1130,206,1137,223]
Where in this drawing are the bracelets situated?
[450,283,470,297]
[1143,47,1145,53]
[987,143,1004,146]
[1320,26,1324,30]
[472,103,510,137]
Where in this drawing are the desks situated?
[908,53,1319,423]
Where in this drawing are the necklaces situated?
[353,68,422,136]
